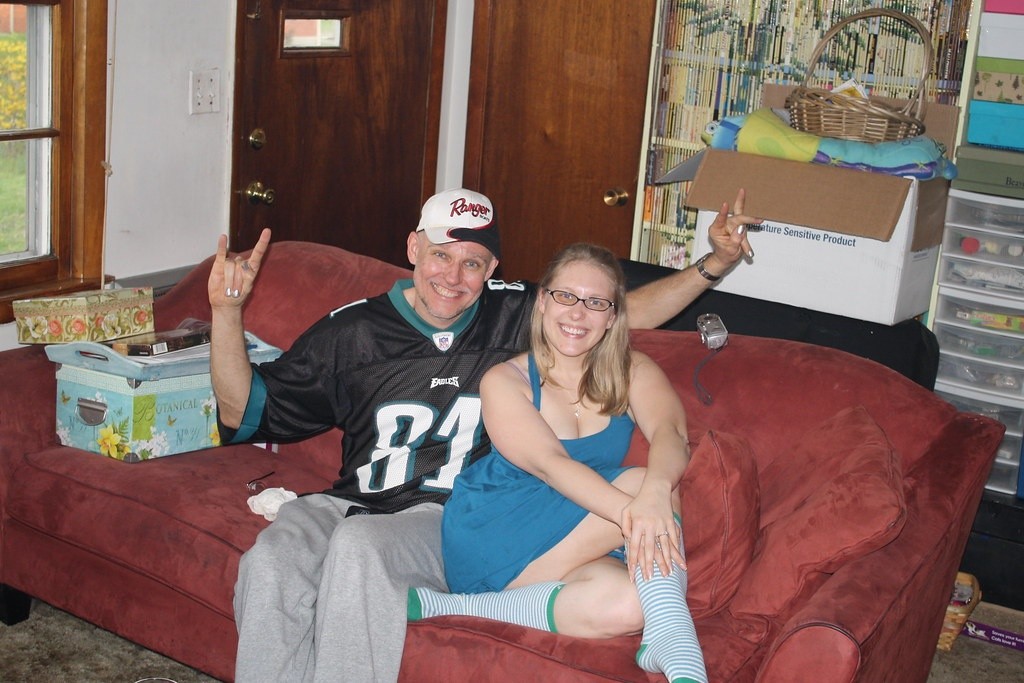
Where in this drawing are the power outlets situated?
[187,68,222,113]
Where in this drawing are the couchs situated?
[0,238,1004,683]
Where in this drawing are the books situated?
[814,77,871,110]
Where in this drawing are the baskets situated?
[784,8,933,144]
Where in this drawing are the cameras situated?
[697,313,728,351]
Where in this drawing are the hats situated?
[416,188,501,261]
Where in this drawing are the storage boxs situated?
[55,365,223,462]
[12,282,155,344]
[690,1,1024,507]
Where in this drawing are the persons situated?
[405,239,707,683]
[208,186,765,683]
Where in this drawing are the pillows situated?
[728,400,907,645]
[674,429,755,618]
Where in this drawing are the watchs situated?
[696,253,720,281]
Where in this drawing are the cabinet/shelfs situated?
[631,1,985,290]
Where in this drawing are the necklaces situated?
[569,389,584,417]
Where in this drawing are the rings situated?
[658,530,669,536]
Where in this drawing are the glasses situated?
[542,284,615,312]
[246,470,275,496]
